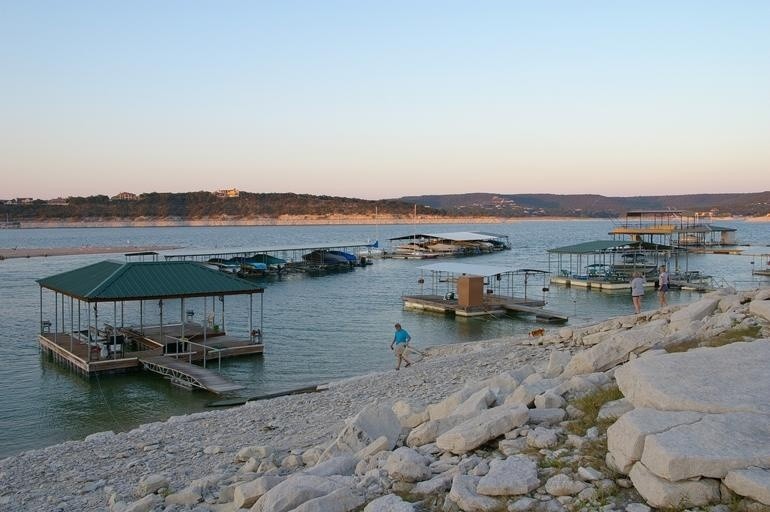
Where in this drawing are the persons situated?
[390,322,413,371]
[656,264,670,308]
[630,271,648,315]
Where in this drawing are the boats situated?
[392,237,506,259]
[613,264,658,275]
[206,248,370,282]
[680,235,703,242]
